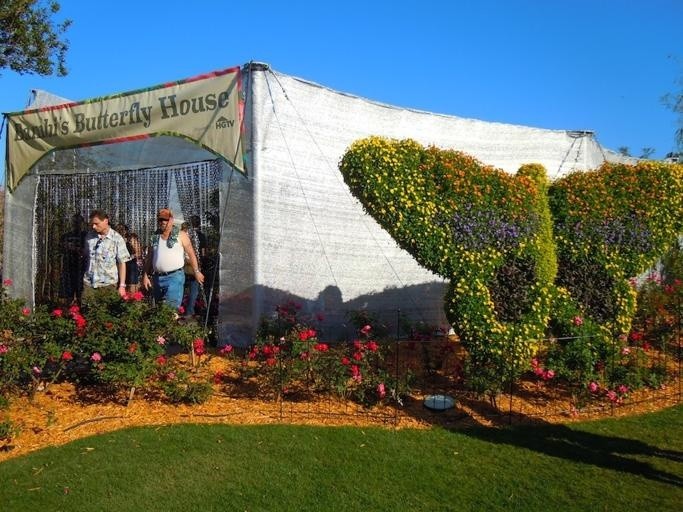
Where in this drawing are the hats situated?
[158,209,172,221]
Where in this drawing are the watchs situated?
[193,269,199,273]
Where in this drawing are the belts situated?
[154,268,182,276]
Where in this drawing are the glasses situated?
[95,238,102,248]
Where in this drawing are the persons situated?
[142,208,206,314]
[113,215,206,313]
[58,215,90,304]
[78,208,128,309]
